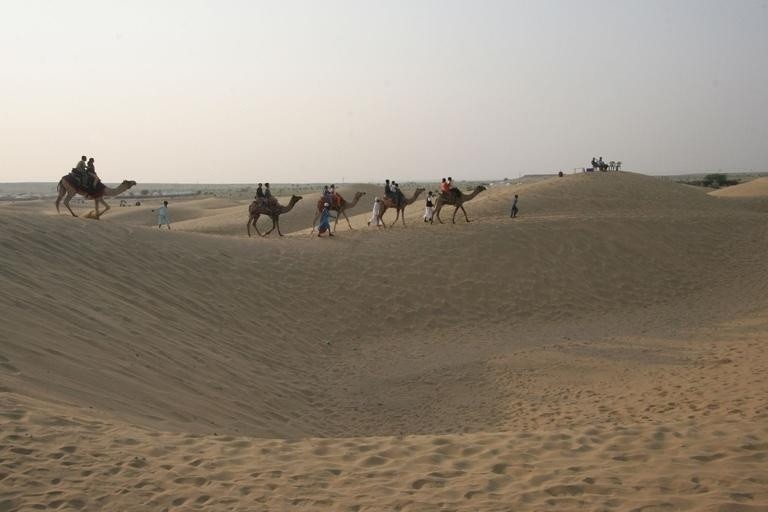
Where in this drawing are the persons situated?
[263,183,272,212]
[366,196,383,227]
[395,183,400,196]
[383,179,396,204]
[329,184,341,206]
[509,194,519,218]
[323,186,332,204]
[85,157,99,192]
[439,177,453,203]
[422,191,435,223]
[316,202,337,237]
[151,200,170,230]
[75,155,89,189]
[597,156,608,171]
[590,157,602,171]
[446,177,456,203]
[389,181,398,196]
[255,183,264,207]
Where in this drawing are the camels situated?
[55,169,136,220]
[376,187,426,230]
[246,194,303,237]
[431,186,487,224]
[310,191,366,234]
